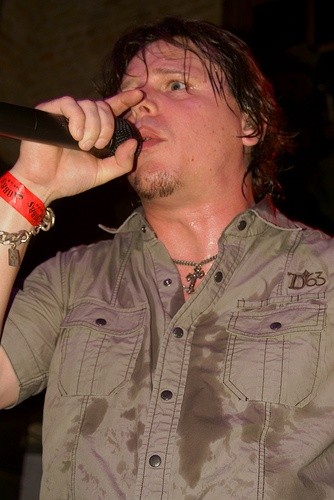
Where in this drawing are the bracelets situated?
[0,171,47,227]
[0,207,55,266]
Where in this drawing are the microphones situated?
[0,102,143,161]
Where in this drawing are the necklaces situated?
[171,254,220,294]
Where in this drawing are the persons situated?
[0,17,334,500]
